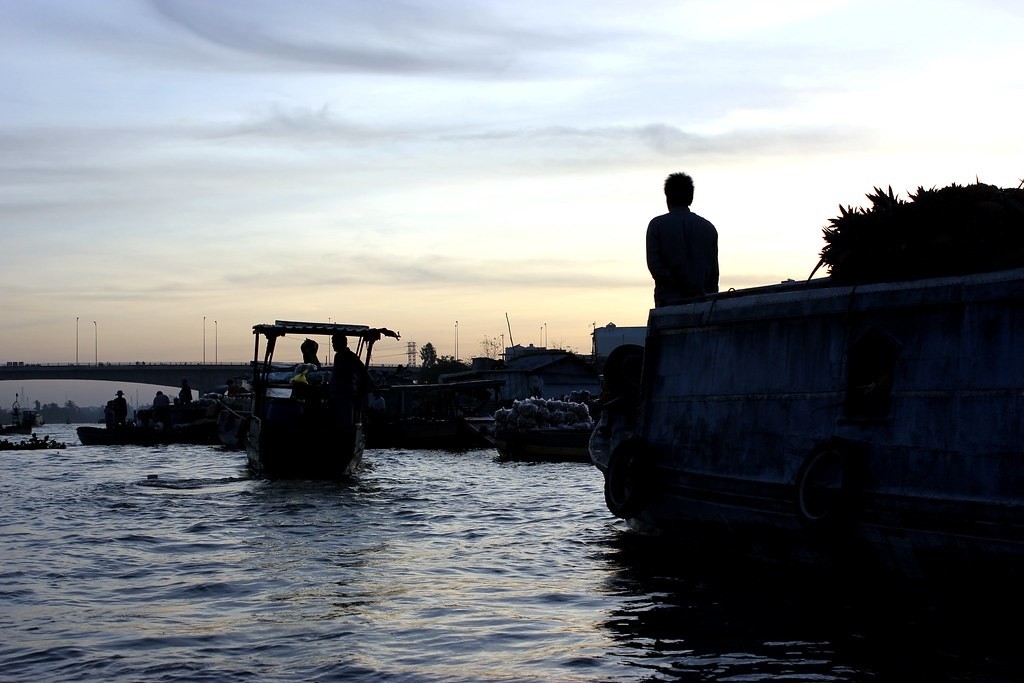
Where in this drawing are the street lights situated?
[93,321,98,365]
[203,317,206,363]
[76,317,79,364]
[215,321,217,363]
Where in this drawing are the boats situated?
[242,319,399,477]
[602,268,1024,563]
[77,425,149,445]
[462,414,592,460]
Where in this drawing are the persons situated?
[288,339,324,424]
[104,400,116,429]
[114,389,128,427]
[152,391,172,429]
[330,333,363,400]
[178,377,194,421]
[225,378,239,395]
[645,172,720,306]
